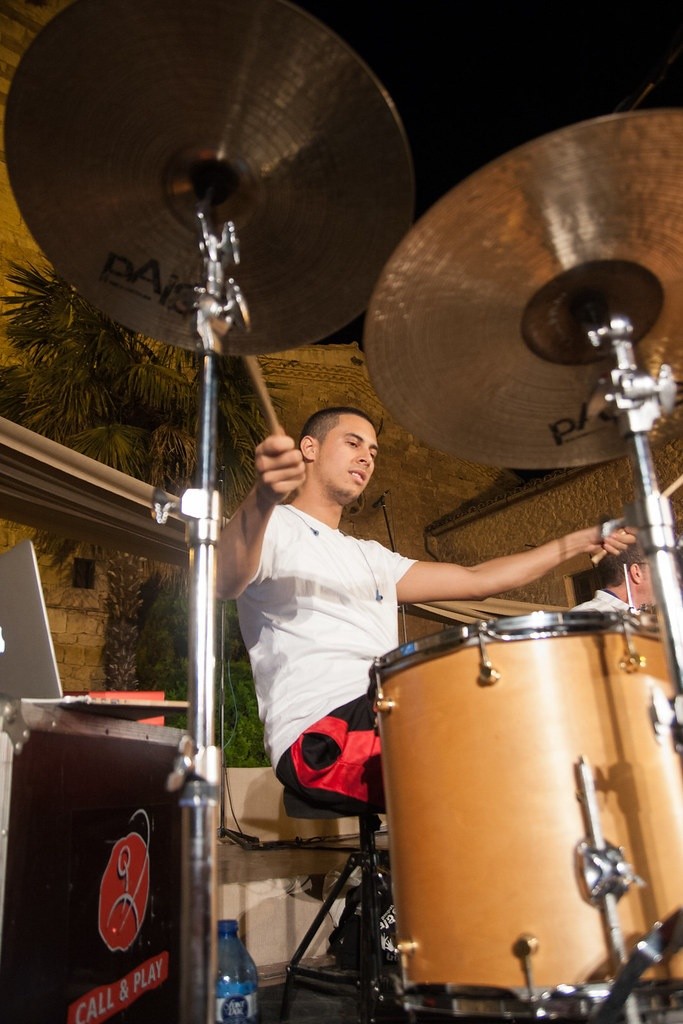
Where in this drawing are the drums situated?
[367,605,683,1019]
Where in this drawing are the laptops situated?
[0,538,190,720]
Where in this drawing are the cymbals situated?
[361,105,683,473]
[2,0,417,361]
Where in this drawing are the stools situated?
[281,788,418,1024]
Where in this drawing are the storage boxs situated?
[0,701,191,1024]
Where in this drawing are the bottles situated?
[214,920,259,1024]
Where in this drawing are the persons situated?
[564,531,658,615]
[211,406,637,813]
